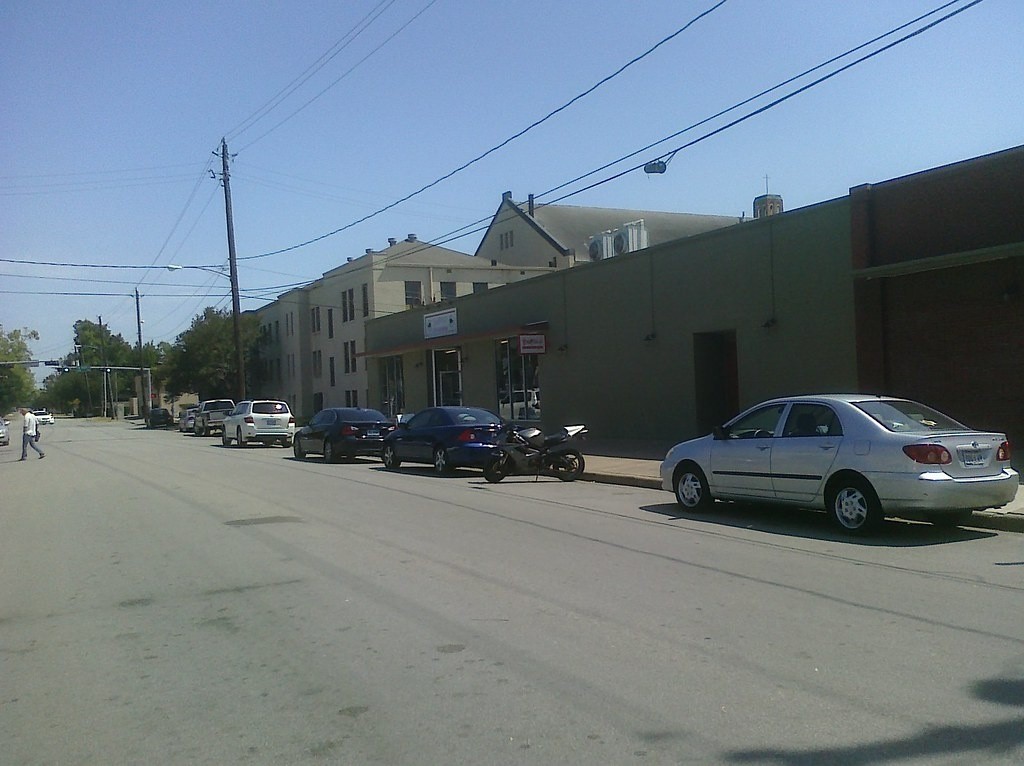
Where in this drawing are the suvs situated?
[221,399,295,448]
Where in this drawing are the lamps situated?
[558,343,568,351]
[762,318,776,330]
[645,333,655,341]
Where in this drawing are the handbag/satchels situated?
[35,432,40,442]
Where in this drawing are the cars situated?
[33,411,55,425]
[660,393,1020,534]
[178,408,199,433]
[0,417,9,445]
[145,408,174,429]
[293,406,396,464]
[379,405,510,475]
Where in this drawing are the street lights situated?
[74,345,115,419]
[168,264,246,401]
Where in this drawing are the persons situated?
[18,408,46,461]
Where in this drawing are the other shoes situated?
[19,458,27,461]
[39,454,46,459]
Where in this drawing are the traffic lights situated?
[151,394,156,398]
[58,368,69,372]
[100,368,111,372]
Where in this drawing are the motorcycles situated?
[481,419,589,483]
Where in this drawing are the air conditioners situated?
[584,232,612,262]
[612,218,649,256]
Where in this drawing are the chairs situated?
[796,413,817,434]
